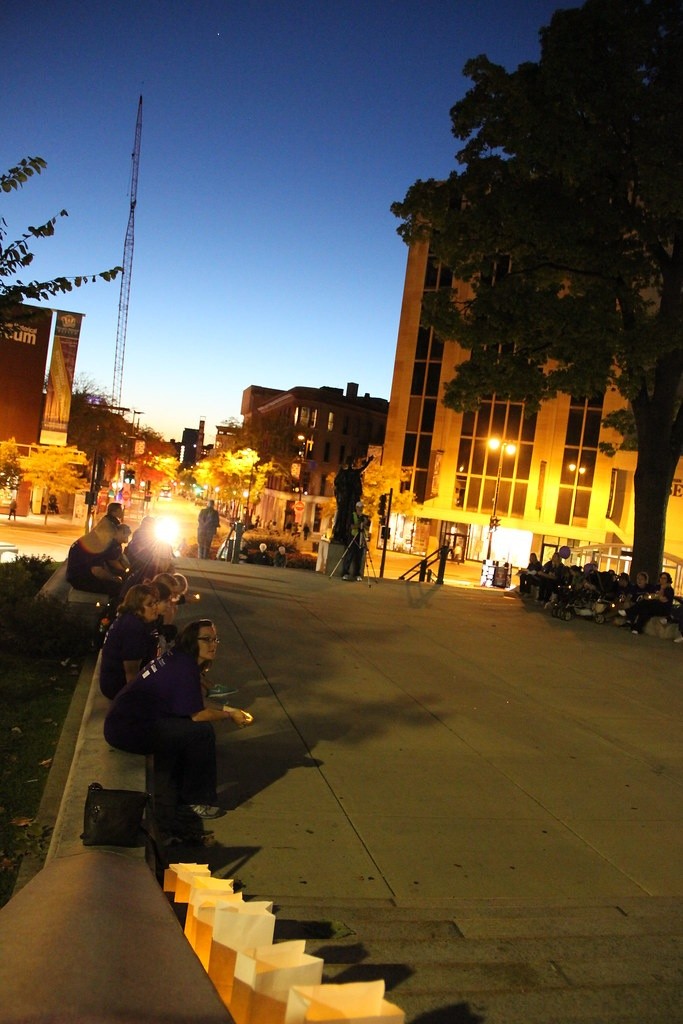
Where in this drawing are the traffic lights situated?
[377,494,387,515]
[490,515,498,531]
[124,469,135,484]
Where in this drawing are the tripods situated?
[329,522,378,588]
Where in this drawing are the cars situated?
[157,487,209,508]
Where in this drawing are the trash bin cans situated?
[312,542,319,553]
[492,561,509,588]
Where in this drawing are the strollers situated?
[551,568,618,625]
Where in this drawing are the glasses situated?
[196,635,220,644]
[141,601,158,607]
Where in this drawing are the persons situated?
[8,498,18,521]
[67,503,176,593]
[216,535,249,564]
[535,552,633,620]
[333,456,374,543]
[454,544,462,565]
[50,498,59,514]
[273,547,287,568]
[254,515,278,531]
[515,552,543,598]
[659,595,683,643]
[197,500,220,560]
[341,502,371,581]
[285,520,301,538]
[99,572,187,698]
[104,617,255,819]
[620,571,657,606]
[303,524,310,539]
[618,572,674,635]
[253,542,273,566]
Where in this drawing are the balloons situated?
[559,546,570,558]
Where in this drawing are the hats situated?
[356,502,364,508]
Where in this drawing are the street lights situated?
[128,410,146,463]
[485,438,517,559]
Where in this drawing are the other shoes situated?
[175,803,222,820]
[354,575,363,581]
[342,574,350,581]
[631,629,640,635]
[618,622,632,630]
[617,609,628,616]
[673,636,683,643]
[656,617,669,626]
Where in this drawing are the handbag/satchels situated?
[80,782,152,847]
